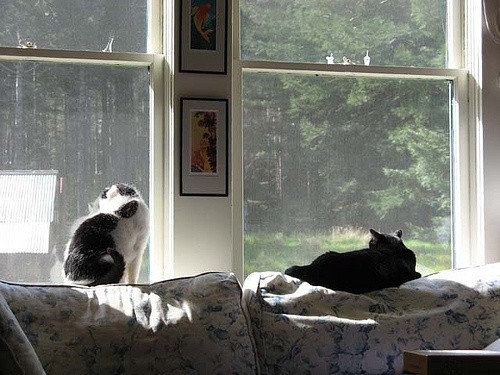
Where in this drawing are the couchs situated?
[0,262,500,374]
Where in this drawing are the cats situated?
[61,184,152,287]
[284,228,421,294]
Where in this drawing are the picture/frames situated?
[179,0,228,73]
[179,97,229,197]
